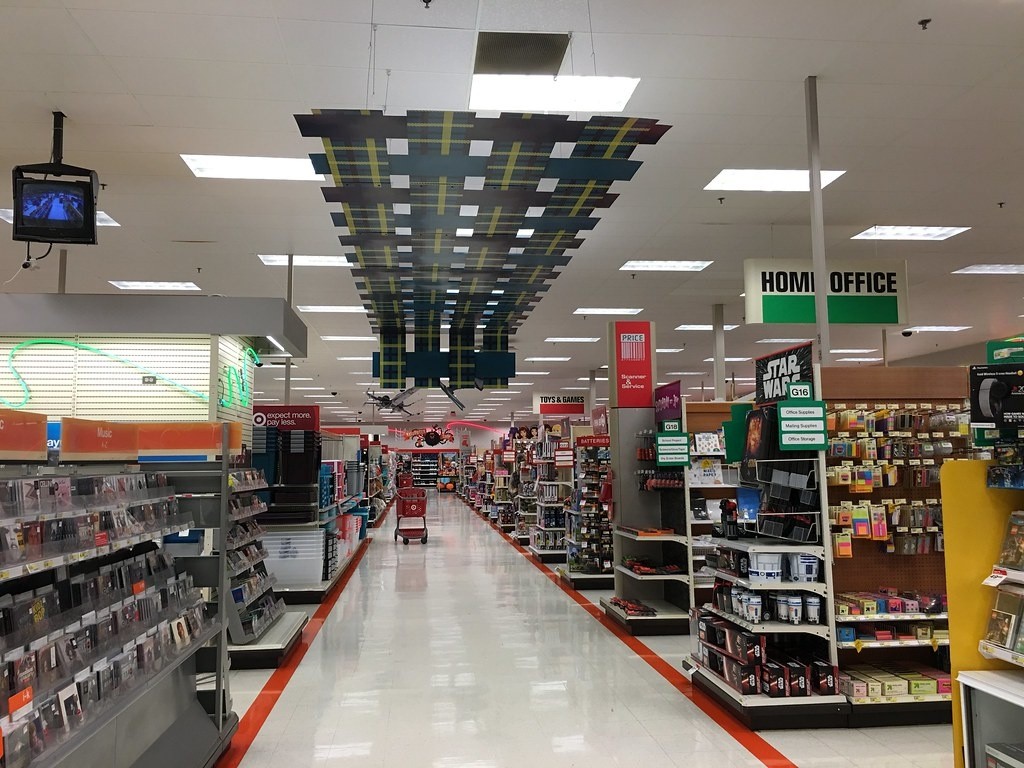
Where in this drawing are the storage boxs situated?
[953,510,1024,768]
[686,589,953,697]
[0,426,369,768]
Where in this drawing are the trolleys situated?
[394,472,429,545]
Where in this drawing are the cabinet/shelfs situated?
[360,434,390,528]
[682,367,977,731]
[527,421,572,564]
[382,444,402,505]
[555,431,615,589]
[600,401,761,636]
[140,461,309,674]
[400,438,535,545]
[0,460,239,768]
[251,404,369,601]
[940,458,1024,768]
